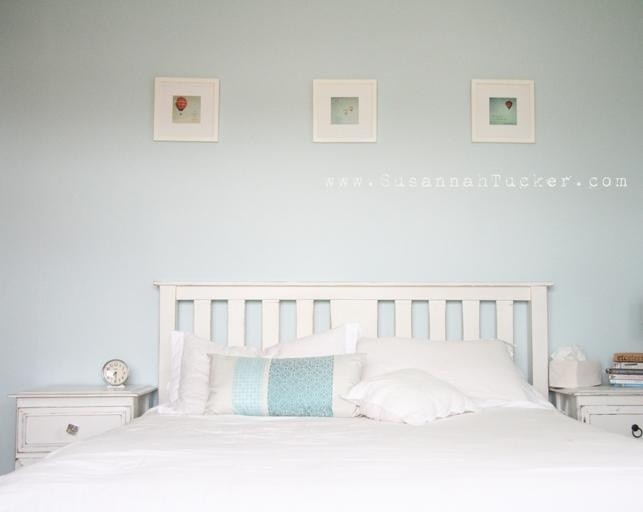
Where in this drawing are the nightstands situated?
[549,387,642,444]
[6,382,158,471]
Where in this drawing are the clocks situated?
[101,358,130,389]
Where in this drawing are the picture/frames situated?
[150,75,220,143]
[310,77,379,144]
[468,78,536,145]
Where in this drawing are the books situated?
[605,353,643,388]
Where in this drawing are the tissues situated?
[548,344,602,389]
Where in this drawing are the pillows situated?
[357,332,551,412]
[203,351,366,422]
[339,364,501,422]
[166,327,364,424]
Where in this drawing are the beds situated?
[13,274,642,471]
